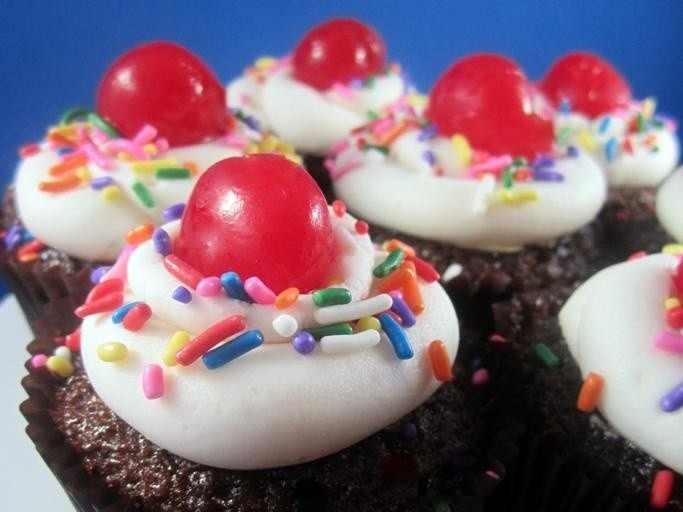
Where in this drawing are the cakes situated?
[623,162,682,258]
[523,51,678,253]
[490,241,683,512]
[225,15,423,204]
[0,42,310,346]
[20,152,480,511]
[323,53,607,326]
